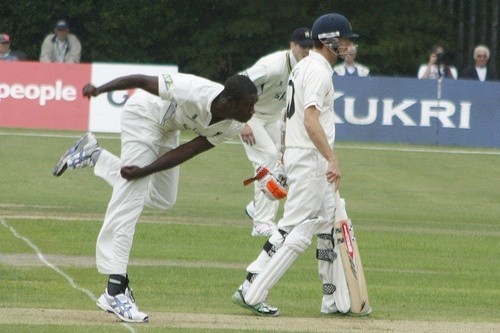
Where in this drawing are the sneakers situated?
[96,288,149,323]
[52,132,100,176]
[231,284,279,316]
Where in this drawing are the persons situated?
[417,44,458,80]
[333,46,370,77]
[232,13,373,316]
[235,27,315,236]
[40,20,81,64]
[460,45,494,82]
[0,34,27,61]
[53,71,259,323]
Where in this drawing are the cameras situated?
[435,51,455,64]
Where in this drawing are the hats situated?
[311,13,359,40]
[0,33,10,43]
[290,27,316,46]
[55,19,69,30]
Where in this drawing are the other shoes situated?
[246,200,277,236]
